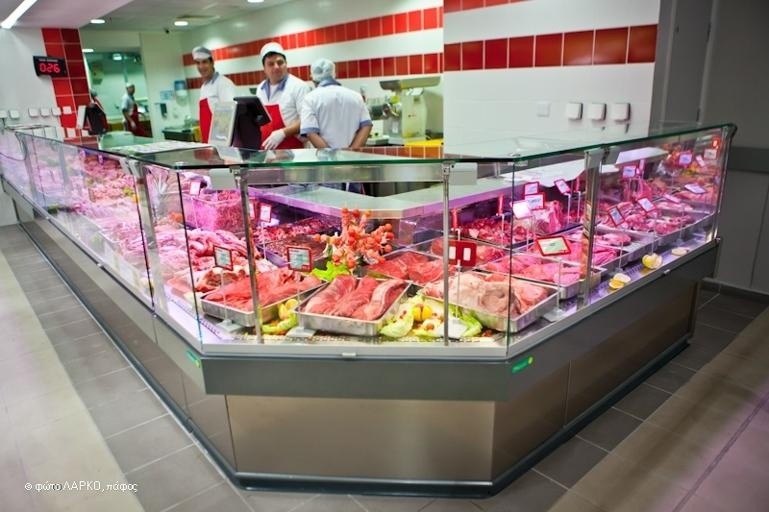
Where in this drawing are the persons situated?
[121,83,147,137]
[192,45,238,143]
[256,41,313,154]
[91,89,112,131]
[300,58,373,195]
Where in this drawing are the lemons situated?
[609,272,631,290]
[642,252,663,270]
[412,304,434,322]
[279,298,297,321]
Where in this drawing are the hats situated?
[126,83,134,88]
[260,42,286,65]
[192,47,211,61]
[310,59,335,81]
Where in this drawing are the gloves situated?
[262,129,285,150]
